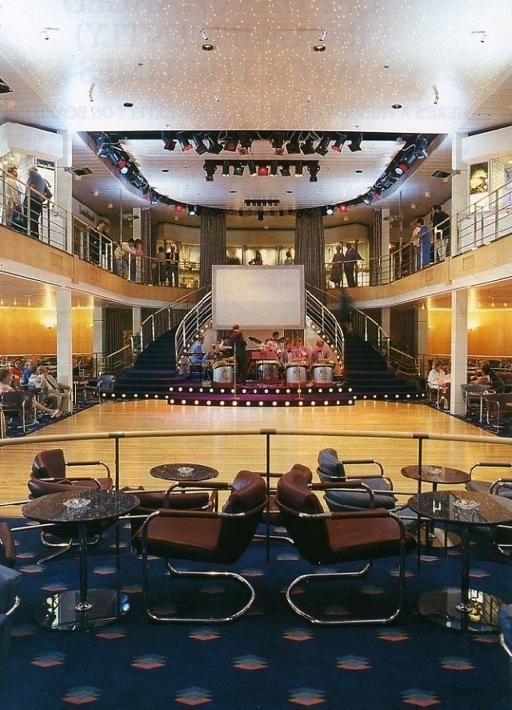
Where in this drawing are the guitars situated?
[202,355,233,369]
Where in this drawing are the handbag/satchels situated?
[12,208,26,231]
[43,185,52,202]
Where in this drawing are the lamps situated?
[57,165,95,177]
[433,87,440,105]
[319,28,328,42]
[431,168,468,179]
[307,94,312,103]
[213,94,220,103]
[199,25,209,40]
[89,85,95,103]
[39,26,50,40]
[481,34,487,43]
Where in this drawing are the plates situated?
[177,467,194,474]
[426,468,441,475]
[63,498,91,508]
[453,499,479,510]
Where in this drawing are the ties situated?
[45,377,56,390]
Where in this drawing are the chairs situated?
[0,374,117,433]
[425,372,512,434]
[185,352,207,381]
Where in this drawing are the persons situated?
[414,217,430,270]
[0,358,62,426]
[23,164,52,240]
[248,252,262,265]
[475,359,504,405]
[428,359,446,408]
[3,166,22,228]
[186,325,331,384]
[328,244,345,288]
[89,218,180,288]
[431,204,450,262]
[407,221,421,273]
[343,243,364,286]
[284,251,292,265]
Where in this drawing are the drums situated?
[213,361,234,384]
[286,360,307,383]
[256,360,280,383]
[312,363,333,385]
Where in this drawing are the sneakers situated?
[49,408,64,419]
